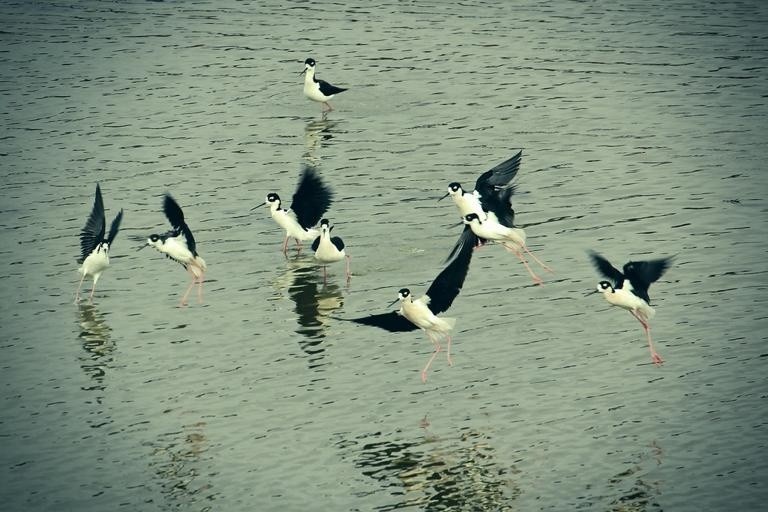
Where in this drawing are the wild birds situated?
[310,215,351,289]
[136,189,208,306]
[581,245,683,366]
[325,231,477,386]
[249,162,338,262]
[297,57,350,113]
[437,146,554,288]
[73,181,123,307]
